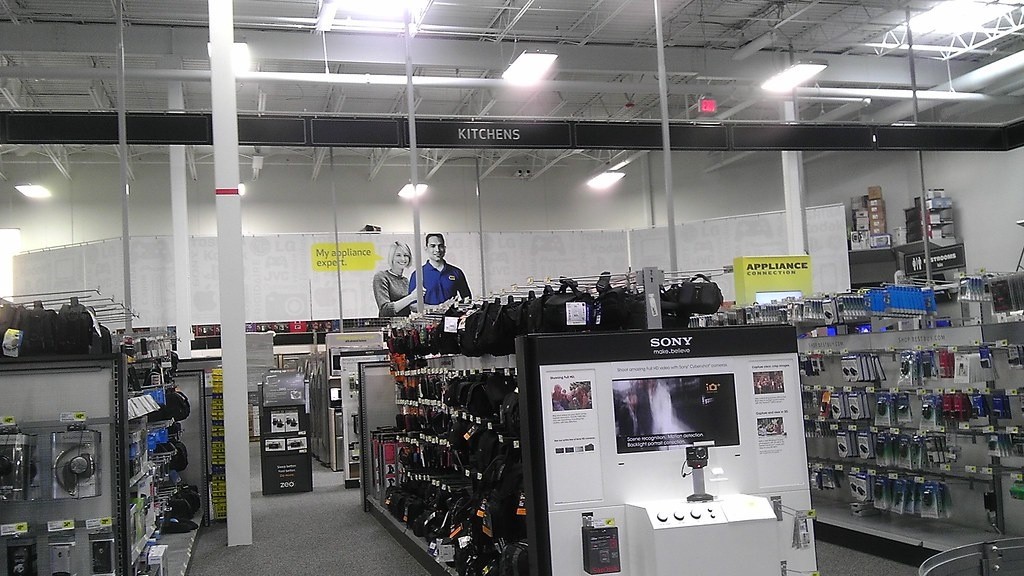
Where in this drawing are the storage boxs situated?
[850,185,892,250]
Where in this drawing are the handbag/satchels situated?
[0,297,112,357]
[386,272,724,576]
[148,388,201,533]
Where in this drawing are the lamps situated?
[762,60,829,92]
[587,171,626,190]
[501,50,558,86]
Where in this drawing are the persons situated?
[552,382,592,410]
[409,233,472,313]
[373,239,426,318]
